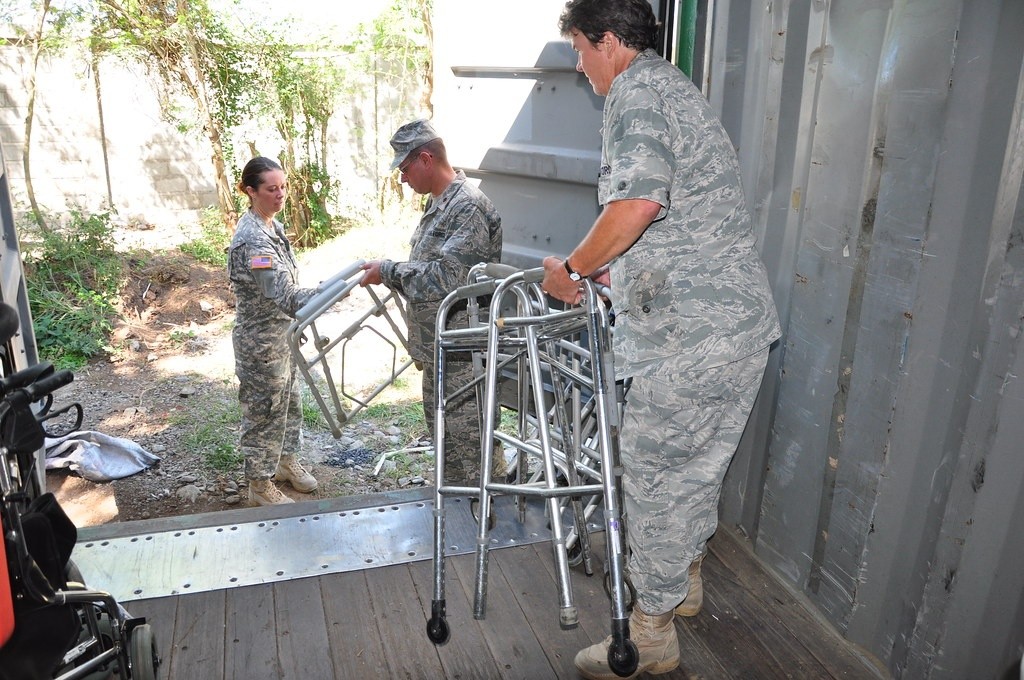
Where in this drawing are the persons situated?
[542,1,784,680]
[227,156,350,507]
[359,119,508,488]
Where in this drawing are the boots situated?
[611,555,704,617]
[573,602,679,680]
[248,481,295,506]
[275,450,318,492]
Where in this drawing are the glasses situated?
[400,151,432,175]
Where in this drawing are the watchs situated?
[563,258,588,281]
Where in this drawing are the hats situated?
[389,118,441,169]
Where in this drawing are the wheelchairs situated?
[0,360,164,680]
